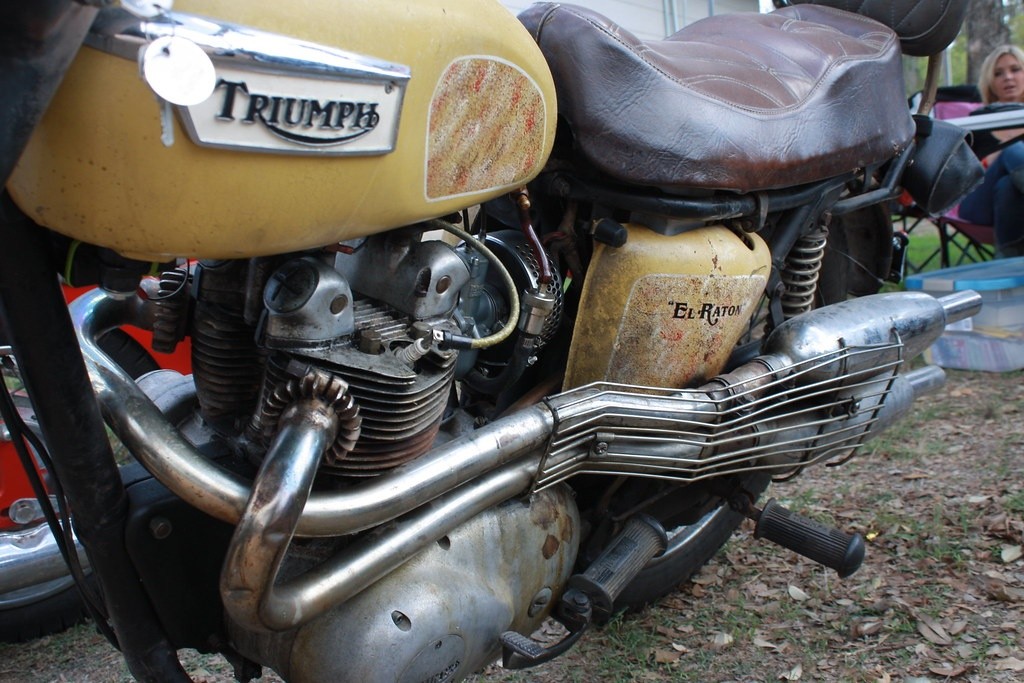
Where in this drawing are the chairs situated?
[895,85,1005,275]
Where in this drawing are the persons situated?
[957,44,1024,257]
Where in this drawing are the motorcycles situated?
[0,0,989,683]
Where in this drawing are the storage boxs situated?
[899,255,1024,374]
